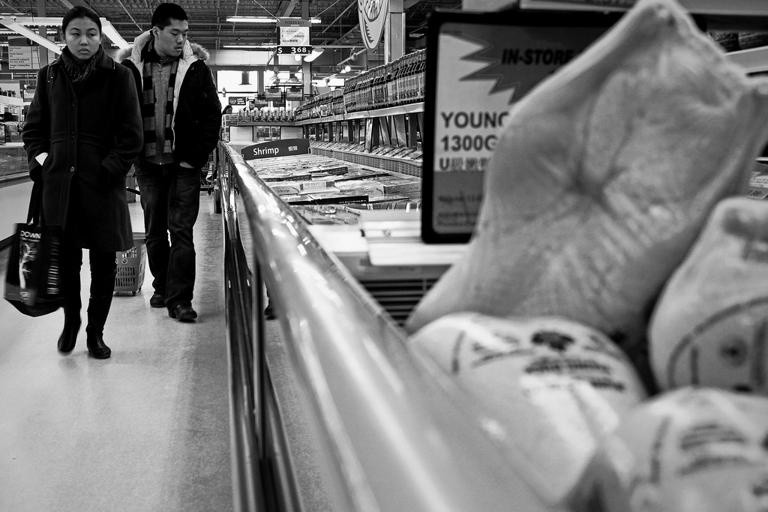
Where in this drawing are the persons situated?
[115,3,222,321]
[24,6,146,358]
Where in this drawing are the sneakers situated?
[165,296,198,322]
[150,291,169,308]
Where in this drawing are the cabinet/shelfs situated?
[0,102,29,181]
[213,46,768,512]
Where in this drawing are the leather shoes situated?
[87,326,113,359]
[57,316,82,355]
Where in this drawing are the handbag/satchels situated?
[2,171,62,318]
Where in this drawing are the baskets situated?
[113,232,150,296]
[126,169,137,204]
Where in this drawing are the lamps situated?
[226,0,322,23]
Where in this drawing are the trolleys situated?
[113,187,148,297]
